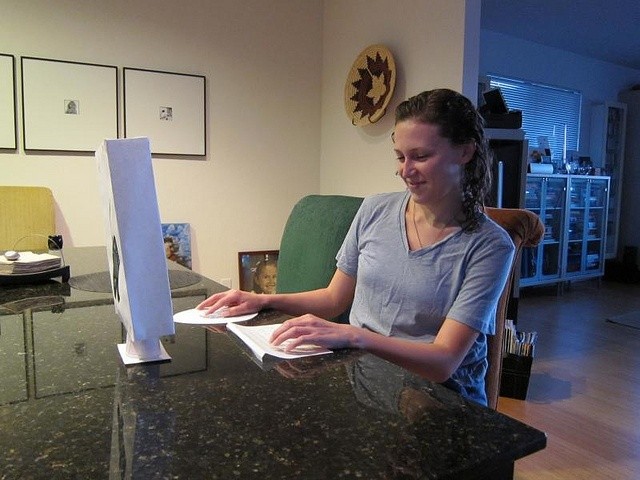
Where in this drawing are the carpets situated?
[607,307,639,330]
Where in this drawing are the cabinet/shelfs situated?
[519,173,611,295]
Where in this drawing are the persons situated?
[164,236,178,261]
[196,88,517,413]
[201,323,481,470]
[252,260,277,294]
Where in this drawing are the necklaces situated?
[412,203,461,256]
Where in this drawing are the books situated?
[504,318,539,356]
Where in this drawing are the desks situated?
[1,246,548,480]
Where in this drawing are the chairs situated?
[275,195,362,323]
[473,206,546,410]
[0,186,56,254]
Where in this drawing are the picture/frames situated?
[123,66,207,156]
[21,56,119,152]
[238,250,279,294]
[0,52,17,150]
